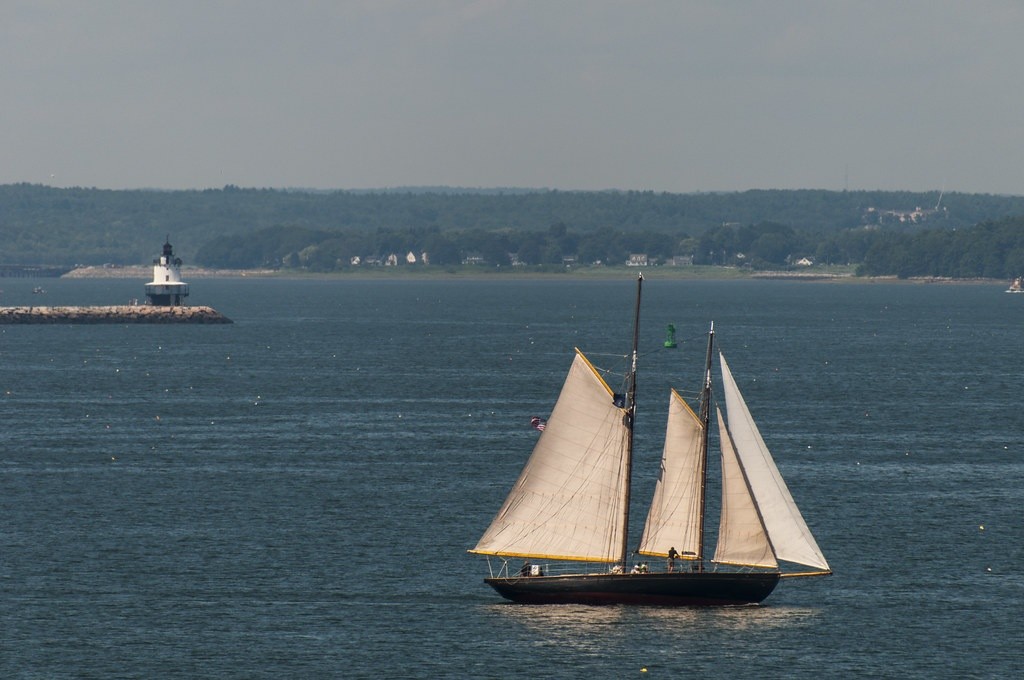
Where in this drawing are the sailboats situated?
[463,268,833,612]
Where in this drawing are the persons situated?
[667,547,679,573]
[631,560,649,574]
[521,561,532,577]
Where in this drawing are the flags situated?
[530,415,547,431]
[612,393,626,409]
